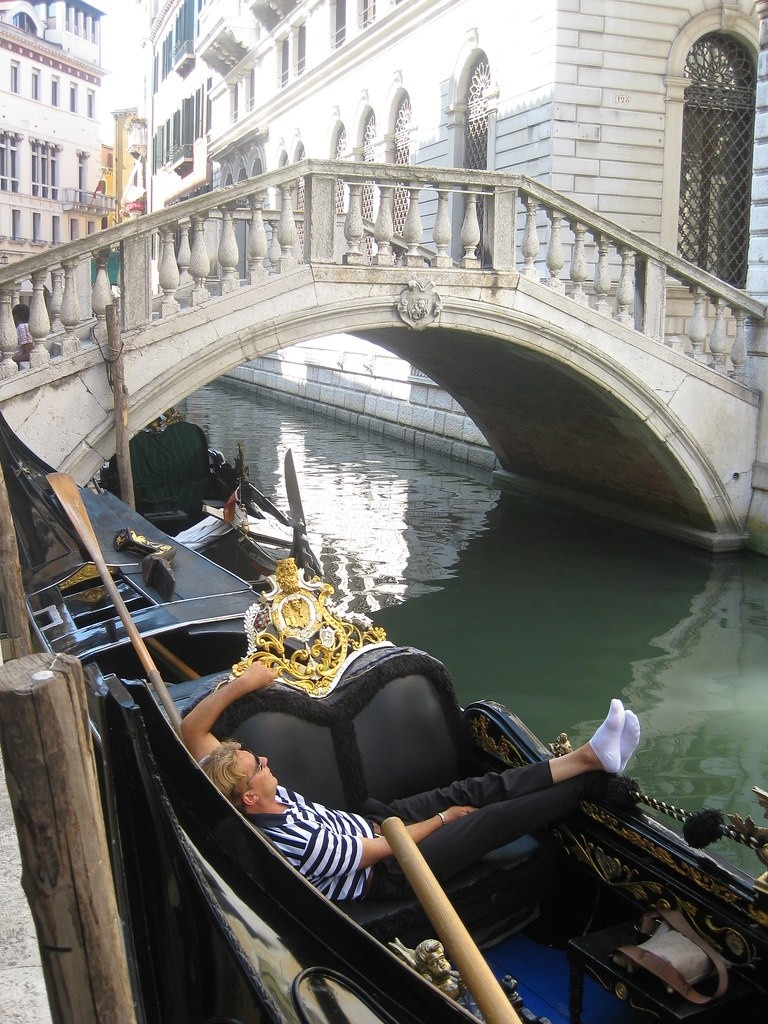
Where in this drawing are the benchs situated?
[185,647,549,956]
[108,422,211,522]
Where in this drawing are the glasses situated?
[238,748,263,784]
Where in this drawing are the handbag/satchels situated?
[608,910,732,1005]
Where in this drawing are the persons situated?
[180,661,642,901]
[12,304,33,362]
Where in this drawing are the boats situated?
[0,408,768,1024]
[85,407,323,600]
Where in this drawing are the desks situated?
[564,915,754,1024]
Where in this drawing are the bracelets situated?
[438,813,447,825]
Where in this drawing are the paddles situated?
[44,471,184,739]
[382,816,520,1024]
